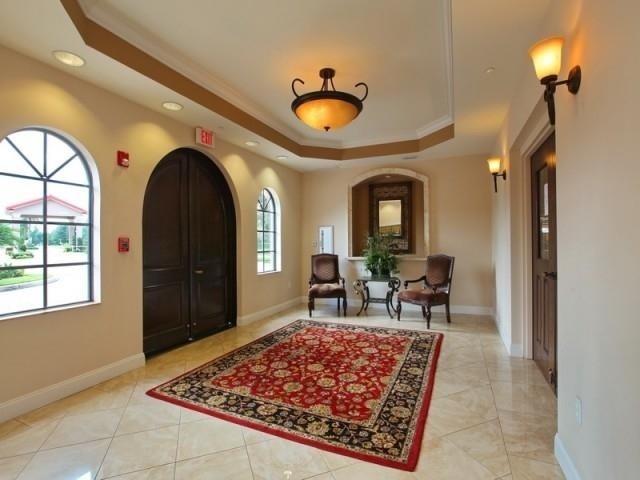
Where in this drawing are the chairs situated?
[397,255,455,328]
[308,253,347,318]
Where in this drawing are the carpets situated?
[146,318,443,472]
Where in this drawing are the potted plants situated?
[361,232,400,278]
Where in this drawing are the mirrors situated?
[376,197,403,238]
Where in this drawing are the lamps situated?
[529,35,581,125]
[487,158,506,192]
[291,68,369,132]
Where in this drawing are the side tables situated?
[354,276,400,319]
